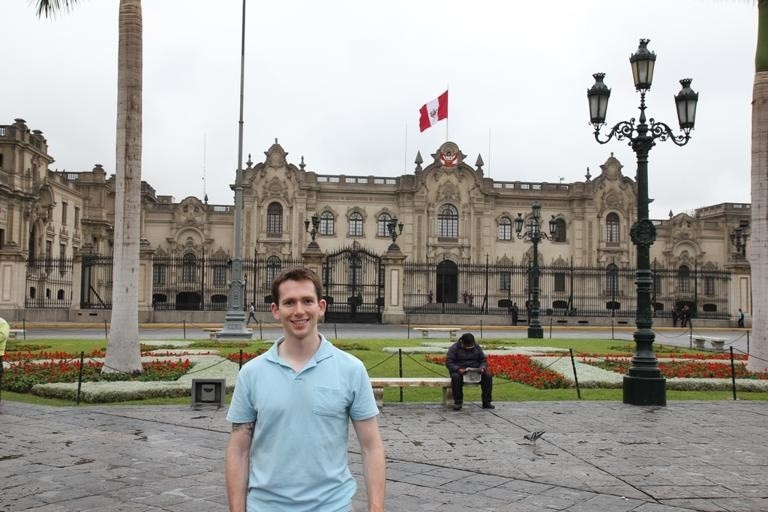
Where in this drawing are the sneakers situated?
[483,403,495,409]
[453,404,462,410]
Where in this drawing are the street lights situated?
[304,215,321,242]
[586,38,699,406]
[730,220,748,254]
[515,201,558,338]
[386,218,404,244]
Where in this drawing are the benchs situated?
[202,327,254,339]
[370,377,462,410]
[413,326,462,341]
[688,334,729,350]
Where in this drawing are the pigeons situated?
[524,430,546,445]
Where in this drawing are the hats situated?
[463,333,474,343]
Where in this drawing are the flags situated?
[419,90,448,132]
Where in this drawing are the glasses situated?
[464,346,474,350]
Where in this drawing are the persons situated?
[225,268,385,512]
[672,304,694,327]
[246,302,259,325]
[446,334,495,410]
[0,316,10,381]
[463,290,474,305]
[509,302,520,326]
[738,309,744,327]
[428,290,433,303]
